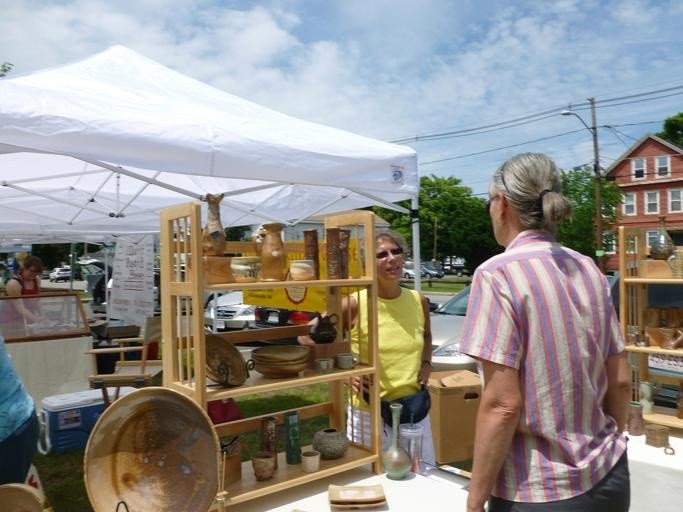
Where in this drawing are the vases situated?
[200,193,228,256]
[627,400,646,435]
[259,223,287,282]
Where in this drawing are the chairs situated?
[84,316,163,409]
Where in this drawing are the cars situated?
[422,269,619,371]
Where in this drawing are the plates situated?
[81,385,222,512]
[205,333,312,387]
[328,484,388,511]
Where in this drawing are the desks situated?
[222,461,489,512]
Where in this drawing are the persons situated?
[466,153,630,512]
[1,252,43,334]
[90,271,112,314]
[297,231,436,468]
[0,335,39,488]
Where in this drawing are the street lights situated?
[558,108,605,274]
[428,204,438,262]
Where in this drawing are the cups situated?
[668,249,683,279]
[643,306,683,350]
[312,352,361,375]
[248,450,322,482]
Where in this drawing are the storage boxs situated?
[426,369,482,465]
[37,386,139,456]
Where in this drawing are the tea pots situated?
[308,310,340,343]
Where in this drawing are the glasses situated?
[376,247,404,259]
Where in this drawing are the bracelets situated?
[422,359,432,366]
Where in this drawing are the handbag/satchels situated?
[363,384,434,428]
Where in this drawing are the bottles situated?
[198,192,352,282]
[676,378,683,419]
[260,410,349,470]
[624,378,655,436]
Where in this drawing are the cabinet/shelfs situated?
[159,201,382,511]
[618,225,682,431]
